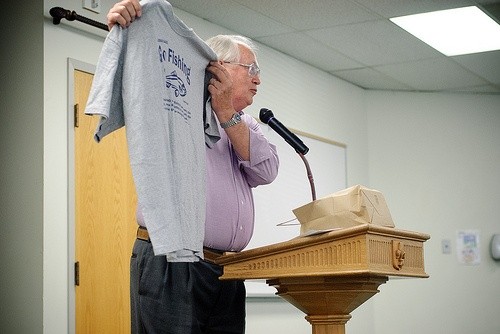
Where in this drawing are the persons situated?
[107,0,279,334]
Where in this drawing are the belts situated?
[137,228,222,265]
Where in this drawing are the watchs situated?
[219,111,242,129]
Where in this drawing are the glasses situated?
[224,61,261,78]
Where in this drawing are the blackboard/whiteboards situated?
[245,116,349,298]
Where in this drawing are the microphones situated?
[259,108,309,154]
